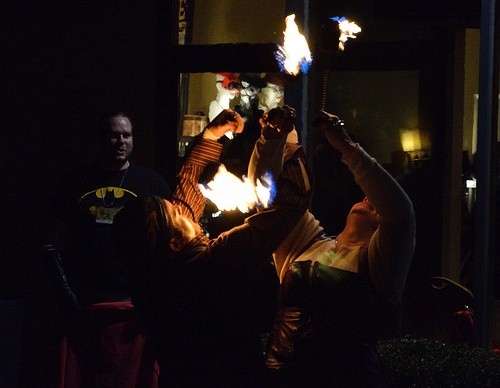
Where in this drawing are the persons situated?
[40,104,417,388]
[208,74,283,139]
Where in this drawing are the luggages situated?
[55,295,162,388]
